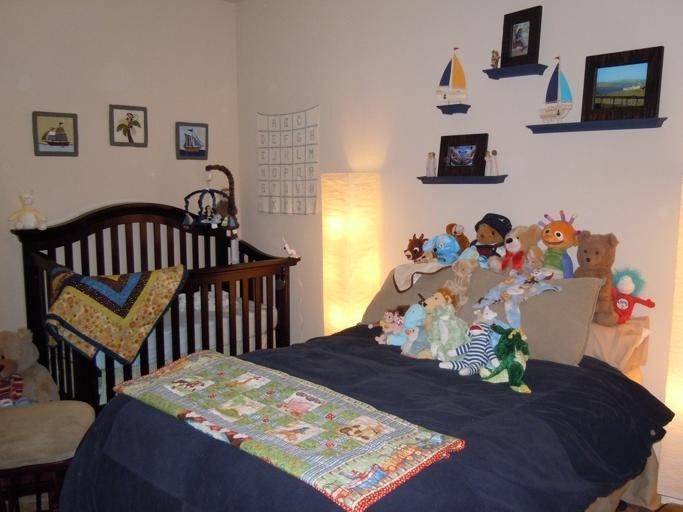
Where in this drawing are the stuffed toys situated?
[186,178,237,237]
[609,266,654,325]
[0,328,60,406]
[574,228,619,326]
[366,211,578,395]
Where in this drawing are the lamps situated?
[316,169,378,334]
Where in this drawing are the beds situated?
[53,213,677,512]
[6,196,300,415]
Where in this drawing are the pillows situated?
[356,262,478,329]
[457,258,609,371]
[585,313,649,379]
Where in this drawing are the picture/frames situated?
[498,3,543,71]
[28,110,81,159]
[577,46,668,124]
[107,102,151,149]
[172,119,211,162]
[435,132,489,178]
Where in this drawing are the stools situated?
[0,393,97,512]
